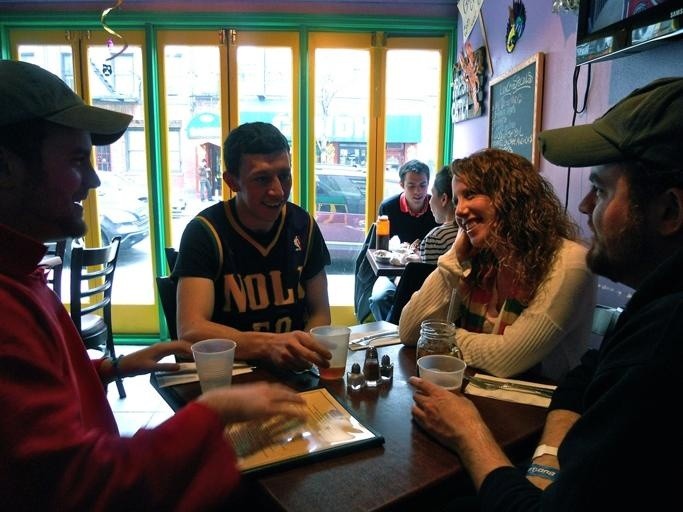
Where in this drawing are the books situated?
[222,382,385,481]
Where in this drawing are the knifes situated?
[472,372,553,392]
[346,332,396,343]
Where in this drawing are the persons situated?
[397,146,597,383]
[384,163,459,327]
[406,74,683,511]
[0,58,313,512]
[197,159,214,202]
[369,159,442,320]
[170,120,333,374]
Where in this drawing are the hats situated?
[537,76,683,184]
[0,59,134,146]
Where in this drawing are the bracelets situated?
[527,463,562,484]
[107,353,126,378]
[530,442,560,462]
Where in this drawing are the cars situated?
[44,172,149,264]
[288,166,403,264]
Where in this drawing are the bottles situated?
[377,354,391,386]
[375,214,389,250]
[415,319,466,376]
[362,346,379,387]
[346,362,363,390]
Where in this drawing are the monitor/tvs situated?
[574,0,683,67]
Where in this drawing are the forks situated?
[463,374,553,401]
[351,332,400,348]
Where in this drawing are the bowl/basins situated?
[374,254,390,263]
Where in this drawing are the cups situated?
[190,339,236,393]
[307,323,349,383]
[416,353,466,395]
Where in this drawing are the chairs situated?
[71,236,126,400]
[391,261,438,324]
[39,237,66,302]
[592,305,622,353]
[156,276,176,341]
